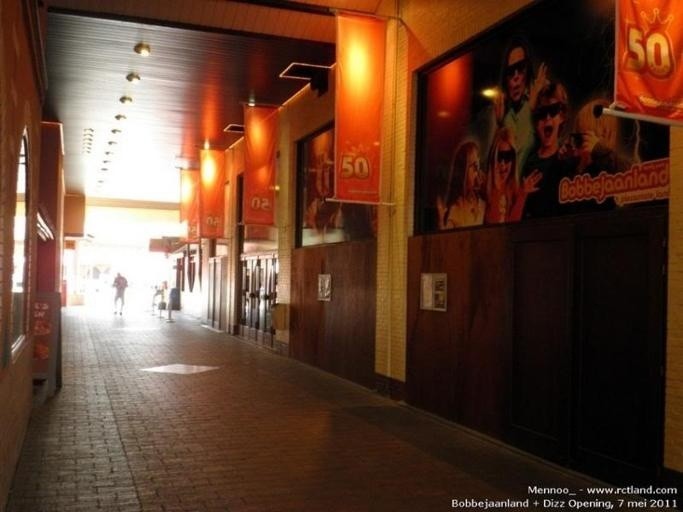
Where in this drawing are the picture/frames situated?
[419,272,449,312]
[316,274,331,302]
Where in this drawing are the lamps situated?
[94,41,152,194]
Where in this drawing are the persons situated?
[159,279,170,320]
[436,139,486,230]
[559,100,628,175]
[477,132,544,224]
[482,37,546,179]
[519,81,573,216]
[113,272,127,315]
